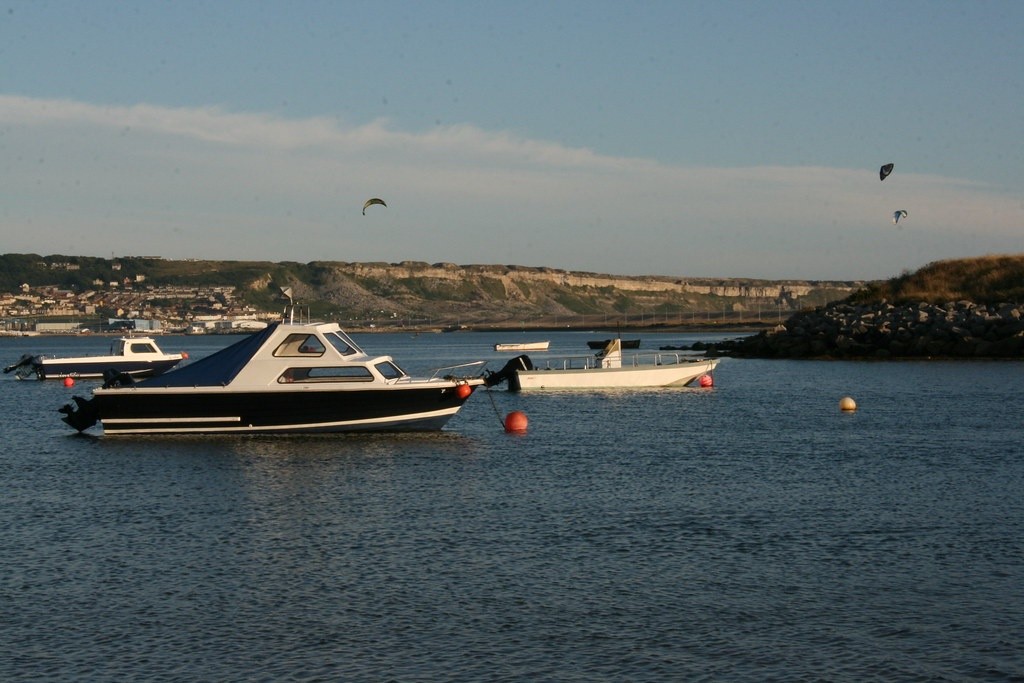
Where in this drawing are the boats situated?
[586,339,640,349]
[4,337,184,382]
[494,341,551,352]
[63,318,489,438]
[183,326,208,335]
[507,341,720,393]
